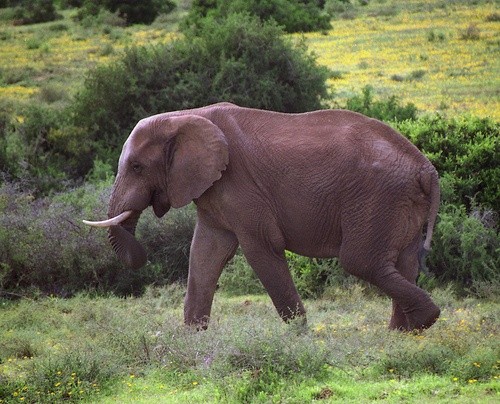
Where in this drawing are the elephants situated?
[81,100,441,336]
